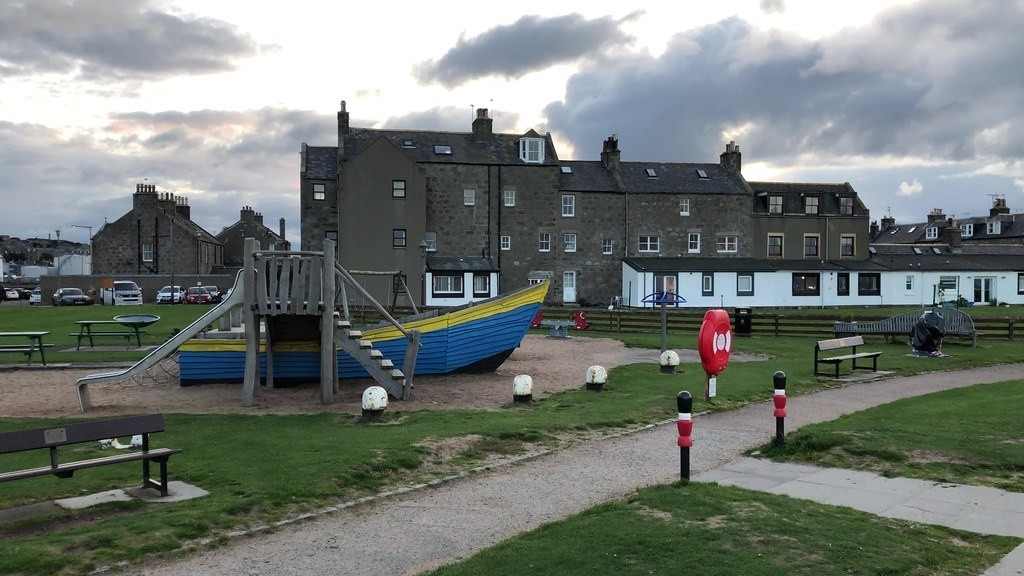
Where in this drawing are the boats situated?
[174,280,552,387]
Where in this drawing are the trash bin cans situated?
[924,310,944,352]
[735,307,752,337]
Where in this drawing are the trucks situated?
[100,280,144,305]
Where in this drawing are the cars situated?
[0,284,42,305]
[203,285,222,304]
[52,287,92,306]
[156,286,186,304]
[183,286,213,305]
[220,288,233,303]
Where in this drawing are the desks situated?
[0,331,51,369]
[73,321,144,352]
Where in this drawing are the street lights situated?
[72,224,92,244]
[55,230,60,276]
[418,240,430,307]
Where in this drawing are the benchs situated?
[0,344,56,348]
[814,336,884,379]
[83,330,148,333]
[0,414,183,496]
[0,349,39,356]
[69,333,136,336]
